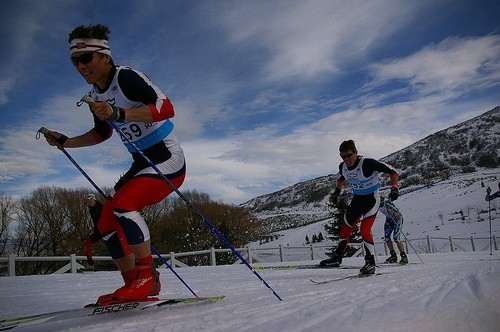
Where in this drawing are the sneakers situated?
[386,250,397,261]
[320,251,342,265]
[97,269,136,306]
[399,252,408,263]
[360,255,375,274]
[114,255,161,299]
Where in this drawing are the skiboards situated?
[0,295,227,332]
[248,262,419,284]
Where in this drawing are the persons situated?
[376,196,409,265]
[43,25,188,308]
[319,139,399,273]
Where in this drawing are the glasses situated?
[340,153,353,159]
[71,48,101,66]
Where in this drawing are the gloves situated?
[329,188,341,204]
[388,188,399,201]
